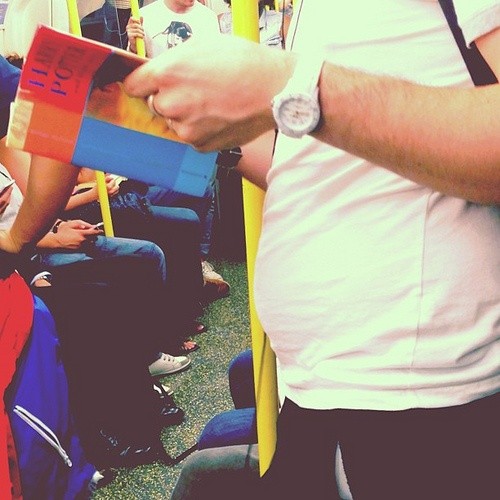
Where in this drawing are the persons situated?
[0,0,500,500]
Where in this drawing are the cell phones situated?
[88,222,104,230]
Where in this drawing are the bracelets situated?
[38,275,51,282]
[52,219,67,233]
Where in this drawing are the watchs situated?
[270,52,324,139]
